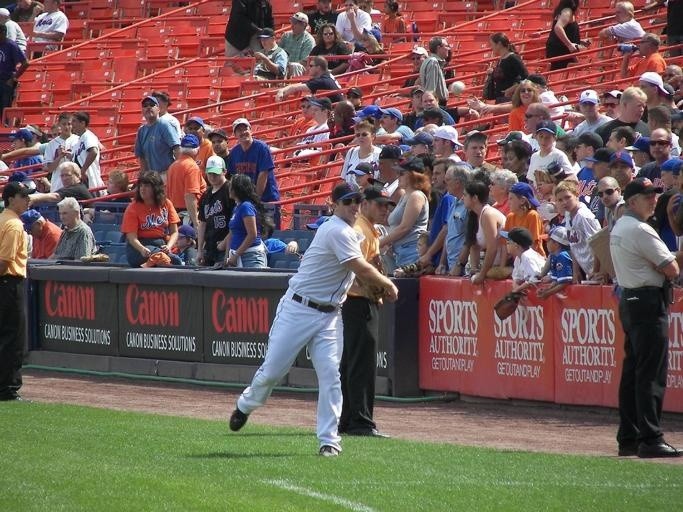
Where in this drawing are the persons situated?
[270,32,683,304]
[227,179,401,461]
[607,175,683,460]
[0,92,279,267]
[597,0,683,59]
[544,2,590,74]
[0,0,71,113]
[0,179,40,406]
[220,0,406,104]
[337,184,403,442]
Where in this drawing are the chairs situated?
[0,0,683,273]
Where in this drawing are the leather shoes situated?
[618,441,683,458]
[346,426,391,438]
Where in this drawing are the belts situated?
[291,292,336,313]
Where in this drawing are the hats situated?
[412,44,428,57]
[306,216,329,230]
[2,126,41,230]
[183,116,250,175]
[631,33,661,45]
[258,28,274,38]
[496,90,682,248]
[301,88,425,121]
[142,96,158,106]
[640,71,667,93]
[331,125,490,206]
[178,224,197,240]
[291,11,309,23]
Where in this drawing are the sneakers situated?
[320,445,338,456]
[229,405,248,432]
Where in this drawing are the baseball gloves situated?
[494,291,527,320]
